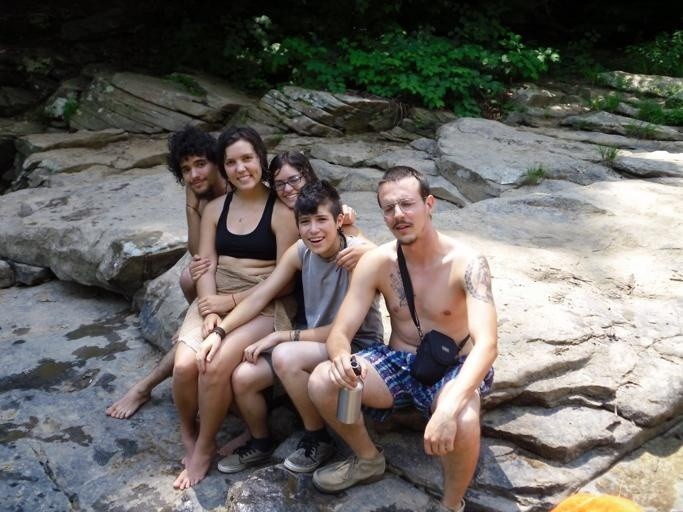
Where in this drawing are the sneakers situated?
[429,498,466,512]
[283,430,336,473]
[312,444,386,495]
[217,440,278,475]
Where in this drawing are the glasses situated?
[380,198,427,216]
[271,174,304,190]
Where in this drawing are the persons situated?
[195,177,387,473]
[172,124,300,491]
[306,165,500,510]
[187,149,357,282]
[106,121,232,418]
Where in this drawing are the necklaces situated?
[224,181,229,193]
[319,235,344,263]
[237,194,247,223]
[339,231,347,249]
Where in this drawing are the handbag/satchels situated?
[409,329,459,386]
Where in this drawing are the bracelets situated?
[208,326,226,341]
[291,329,301,341]
[187,203,198,211]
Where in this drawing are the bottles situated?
[336,360,364,425]
